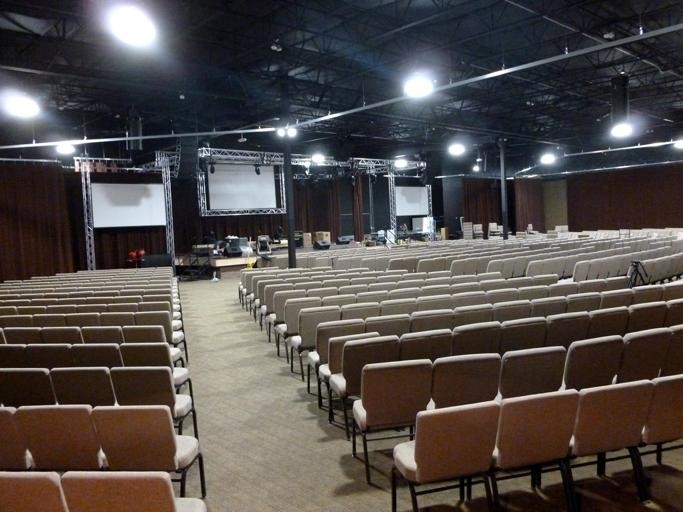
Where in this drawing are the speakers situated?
[336,237,349,244]
[177,128,197,182]
[423,153,438,184]
[223,247,242,256]
[313,239,330,249]
[192,247,210,258]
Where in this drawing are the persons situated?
[275,225,284,239]
[206,229,215,243]
[399,223,408,232]
[255,229,261,241]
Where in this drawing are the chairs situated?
[267,296,683,512]
[239,227,682,316]
[0,261,209,512]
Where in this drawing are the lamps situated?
[604,71,640,142]
